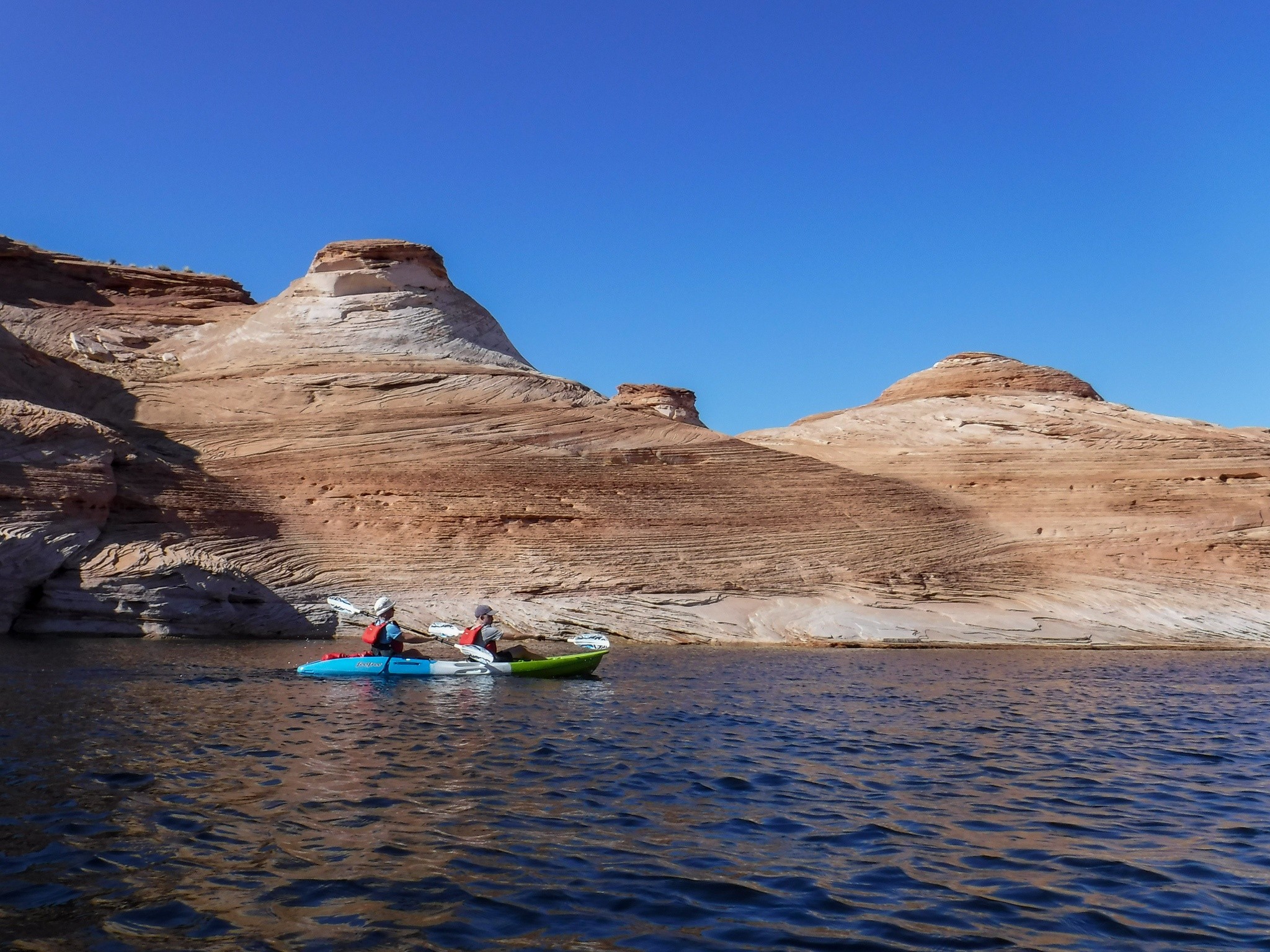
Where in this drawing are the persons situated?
[361,596,438,660]
[460,604,547,663]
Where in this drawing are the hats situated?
[475,604,499,617]
[374,597,396,617]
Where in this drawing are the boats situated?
[297,649,610,678]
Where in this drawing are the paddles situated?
[428,622,610,650]
[327,596,494,665]
